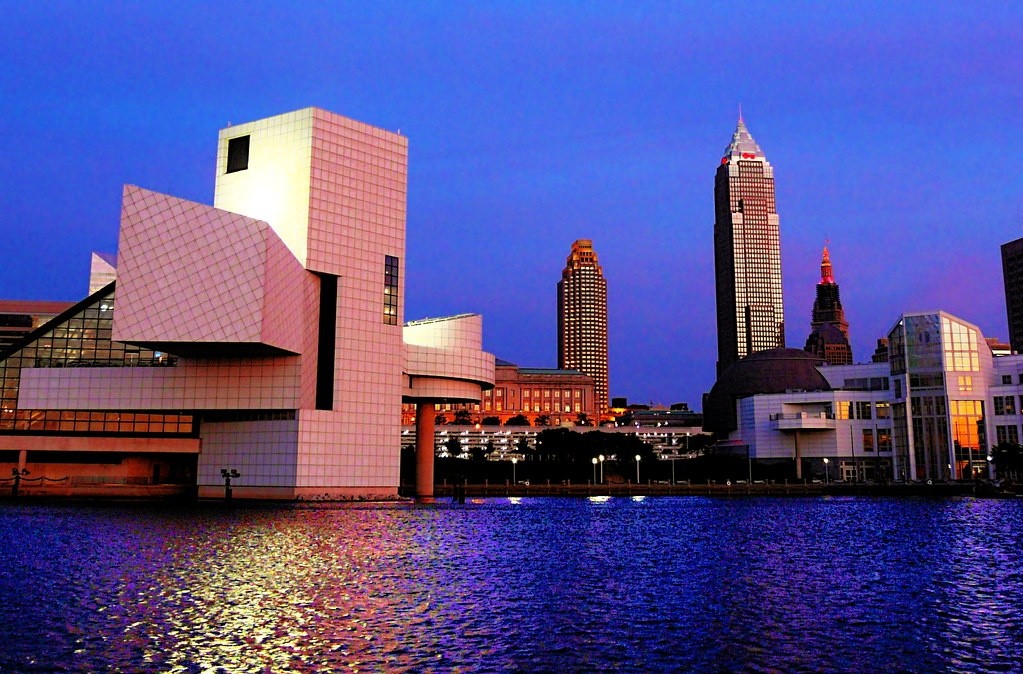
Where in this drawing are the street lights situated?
[512,457,518,485]
[599,455,604,484]
[591,458,597,485]
[823,458,828,483]
[635,455,640,484]
